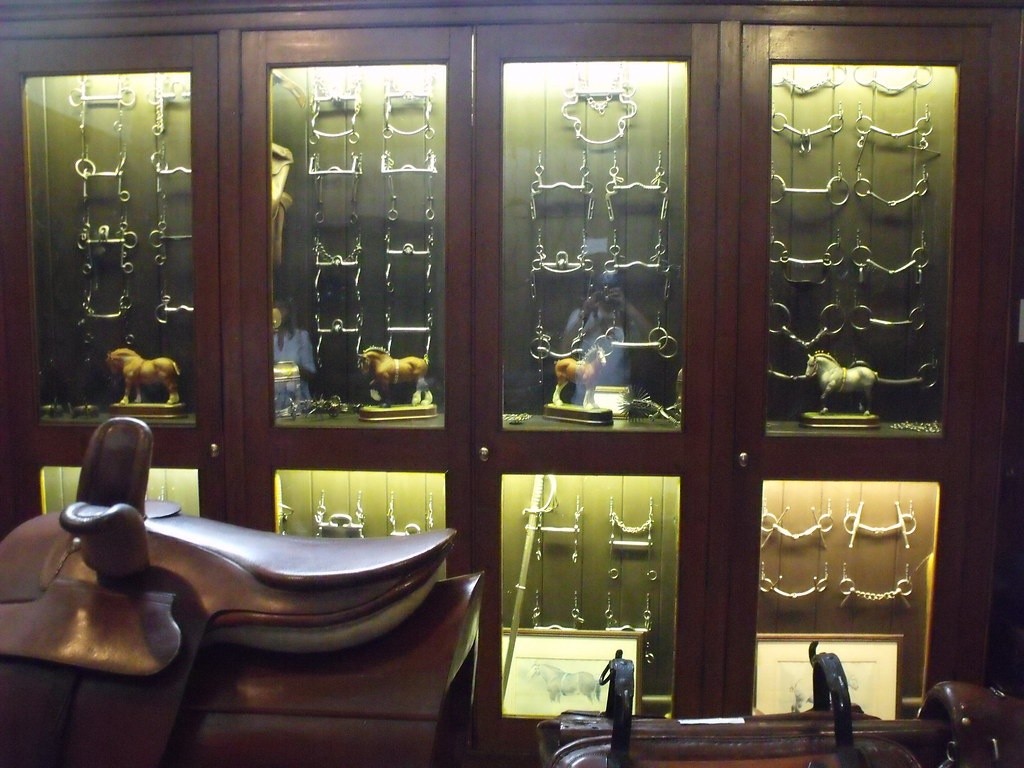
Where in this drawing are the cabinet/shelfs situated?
[237,24,473,580]
[479,22,710,752]
[721,22,988,717]
[0,33,227,539]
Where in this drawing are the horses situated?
[105,348,180,405]
[551,345,607,410]
[356,345,433,406]
[804,350,879,416]
